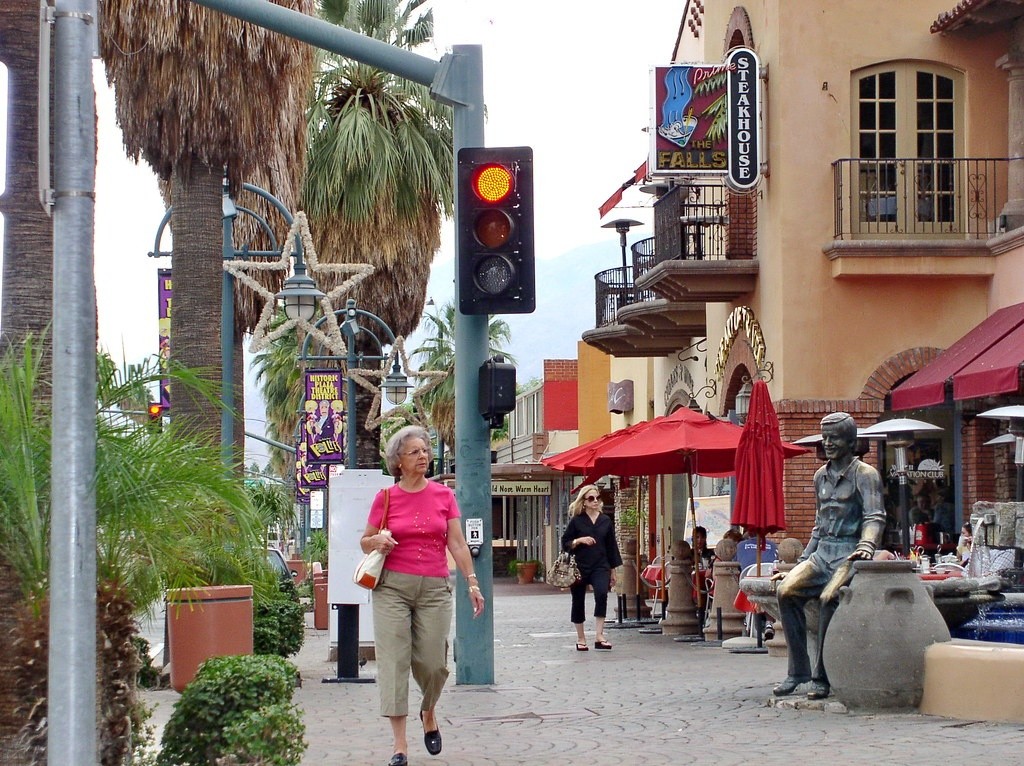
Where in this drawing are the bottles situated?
[709,556,715,570]
[781,561,786,573]
[773,560,780,575]
[915,523,927,546]
[922,555,930,574]
[886,514,903,546]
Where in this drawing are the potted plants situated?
[507,559,543,584]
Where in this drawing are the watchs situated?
[466,573,477,581]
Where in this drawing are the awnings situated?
[890,301,1024,411]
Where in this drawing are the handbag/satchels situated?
[353,487,392,590]
[545,550,582,591]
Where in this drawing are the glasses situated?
[584,495,603,502]
[400,447,430,455]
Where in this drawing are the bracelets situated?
[573,539,576,546]
[468,586,480,592]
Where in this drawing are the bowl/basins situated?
[936,568,945,574]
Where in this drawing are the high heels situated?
[576,643,588,651]
[595,639,612,649]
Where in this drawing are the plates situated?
[931,570,951,574]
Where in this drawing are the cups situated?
[895,557,906,561]
[910,556,920,568]
[938,531,950,544]
[935,554,942,564]
[701,557,709,570]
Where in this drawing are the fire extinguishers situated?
[914,522,926,544]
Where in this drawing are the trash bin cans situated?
[166,584,253,693]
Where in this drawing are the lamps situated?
[735,363,775,423]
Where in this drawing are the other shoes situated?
[419,710,442,755]
[764,625,775,642]
[387,752,408,766]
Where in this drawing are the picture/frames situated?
[912,437,942,467]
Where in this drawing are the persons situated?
[774,411,886,699]
[883,462,955,539]
[705,530,744,578]
[687,526,715,570]
[957,523,973,570]
[732,529,779,641]
[561,484,623,652]
[360,426,485,766]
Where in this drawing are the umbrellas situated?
[730,380,784,654]
[542,407,812,643]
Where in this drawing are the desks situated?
[642,565,711,620]
[678,214,735,260]
